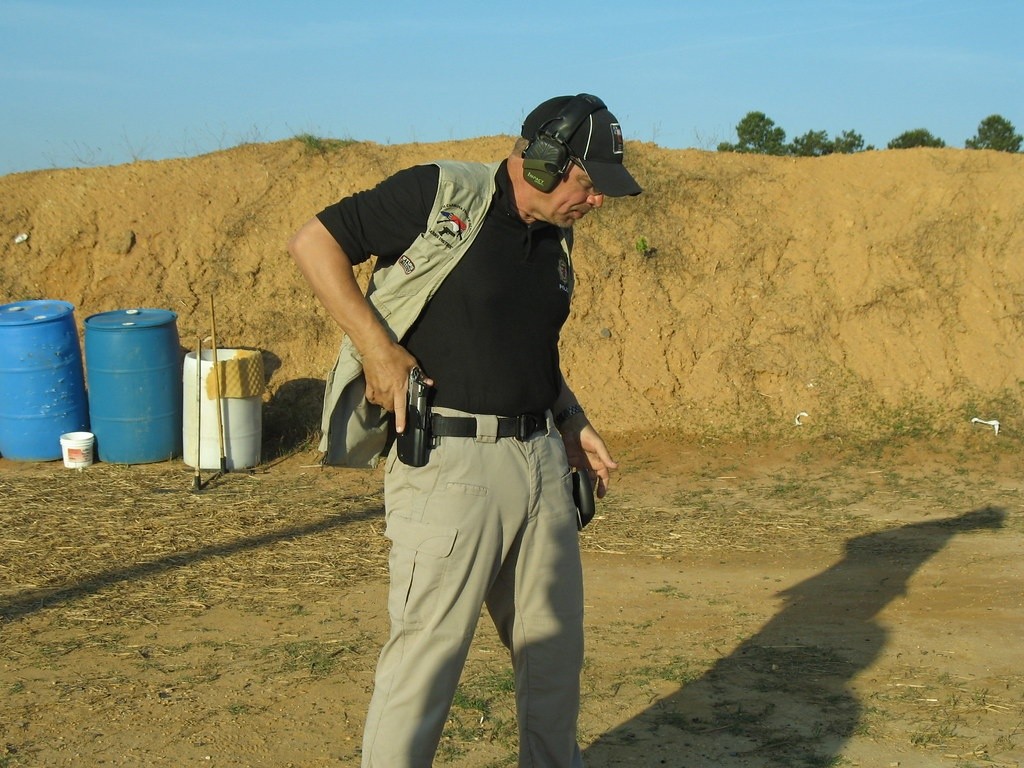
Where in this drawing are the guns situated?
[396,366,435,468]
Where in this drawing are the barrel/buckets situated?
[59,431,96,469]
[84,308,187,463]
[179,347,264,470]
[1,300,87,463]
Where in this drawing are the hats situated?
[520,95,644,197]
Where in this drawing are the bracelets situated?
[555,404,584,419]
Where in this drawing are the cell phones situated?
[406,377,427,430]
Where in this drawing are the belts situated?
[429,409,554,441]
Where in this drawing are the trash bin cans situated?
[182,349,265,470]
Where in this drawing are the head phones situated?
[520,93,608,194]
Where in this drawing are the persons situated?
[287,91,644,768]
